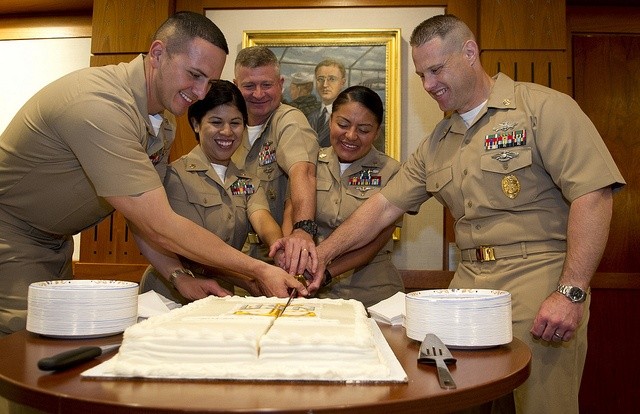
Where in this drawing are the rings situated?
[554,333,562,338]
[302,249,308,252]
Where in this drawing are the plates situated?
[80,317,410,384]
[406,289,513,350]
[26,279,139,338]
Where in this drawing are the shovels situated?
[415,332,458,389]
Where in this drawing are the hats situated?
[290,72,315,84]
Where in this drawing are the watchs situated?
[291,219,317,237]
[322,269,331,287]
[169,267,195,285]
[556,284,587,302]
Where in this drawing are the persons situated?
[276,85,405,316]
[308,61,346,150]
[140,79,299,306]
[0,9,308,337]
[233,48,319,277]
[282,73,318,133]
[300,15,628,414]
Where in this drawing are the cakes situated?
[104,296,394,379]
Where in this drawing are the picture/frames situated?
[241,28,402,242]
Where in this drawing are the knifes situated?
[37,344,122,371]
[279,275,309,318]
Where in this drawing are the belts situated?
[461,240,567,262]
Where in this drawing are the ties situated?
[316,106,330,135]
